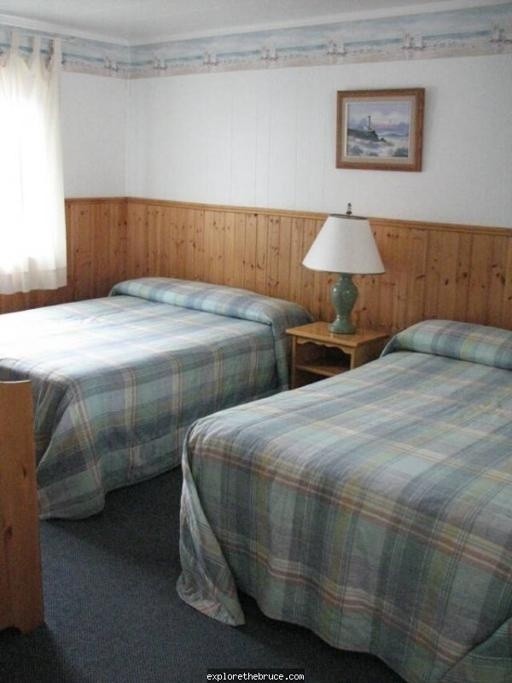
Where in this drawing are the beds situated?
[0,275,312,522]
[175,317,511,682]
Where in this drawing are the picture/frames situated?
[335,86,424,172]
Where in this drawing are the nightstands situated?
[286,320,389,391]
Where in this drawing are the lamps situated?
[301,205,388,333]
